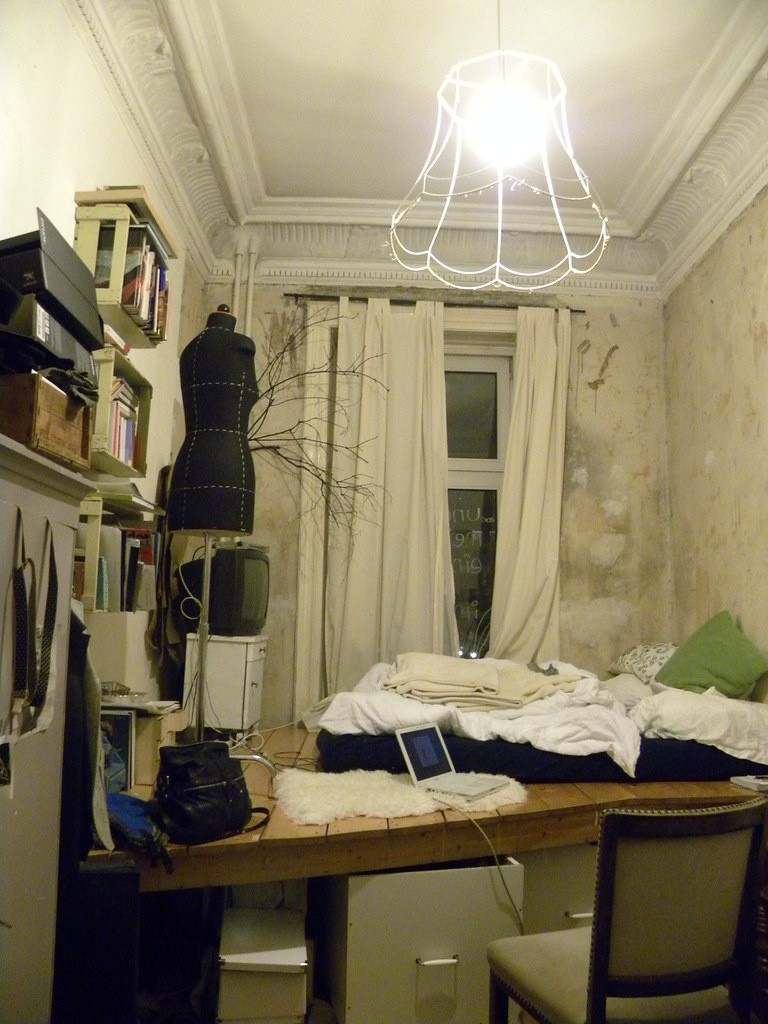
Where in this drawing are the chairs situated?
[491,794,768,1024]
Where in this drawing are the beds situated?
[130,650,768,894]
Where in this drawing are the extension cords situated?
[214,732,244,748]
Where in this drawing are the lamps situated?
[385,2,610,291]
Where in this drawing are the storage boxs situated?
[0,205,106,472]
[216,843,596,1024]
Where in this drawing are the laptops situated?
[395,721,511,804]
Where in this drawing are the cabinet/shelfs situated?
[71,182,180,478]
[182,630,270,747]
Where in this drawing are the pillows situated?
[607,642,682,685]
[653,611,768,700]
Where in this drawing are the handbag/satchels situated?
[152,742,252,843]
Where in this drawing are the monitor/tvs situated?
[175,548,271,638]
